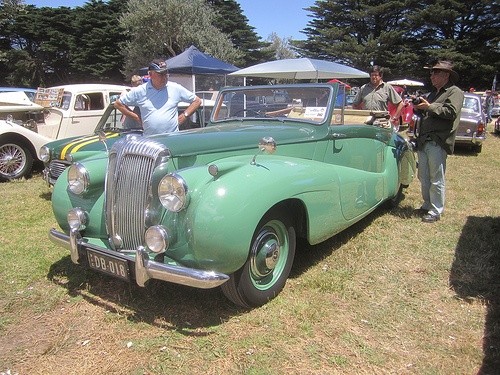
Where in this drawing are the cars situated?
[49,82,420,307]
[0,82,500,186]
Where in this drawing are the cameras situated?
[412,95,427,113]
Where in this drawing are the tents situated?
[140,44,248,123]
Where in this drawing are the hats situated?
[423,61,459,84]
[149,60,170,73]
[470,88,476,92]
[487,90,491,93]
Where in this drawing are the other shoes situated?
[414,208,429,215]
[489,119,493,123]
[422,213,439,223]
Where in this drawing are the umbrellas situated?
[386,79,424,86]
[228,57,371,80]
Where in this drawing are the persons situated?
[352,61,495,224]
[114,62,202,137]
